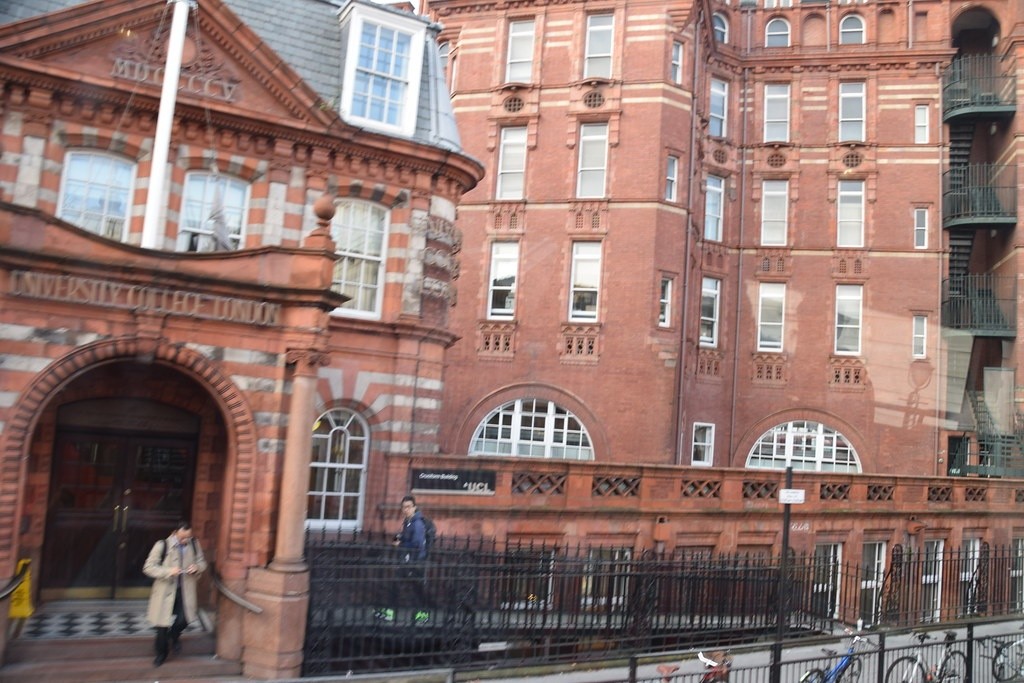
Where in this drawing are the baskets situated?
[845,636,881,659]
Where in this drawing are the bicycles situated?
[991,623,1024,683]
[796,626,882,683]
[656,648,731,683]
[883,631,969,683]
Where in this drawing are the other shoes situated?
[154,650,169,667]
[415,610,429,623]
[168,632,182,653]
[372,608,394,622]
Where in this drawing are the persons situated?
[370,496,429,628]
[141,518,207,667]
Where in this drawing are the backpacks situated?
[422,518,438,560]
